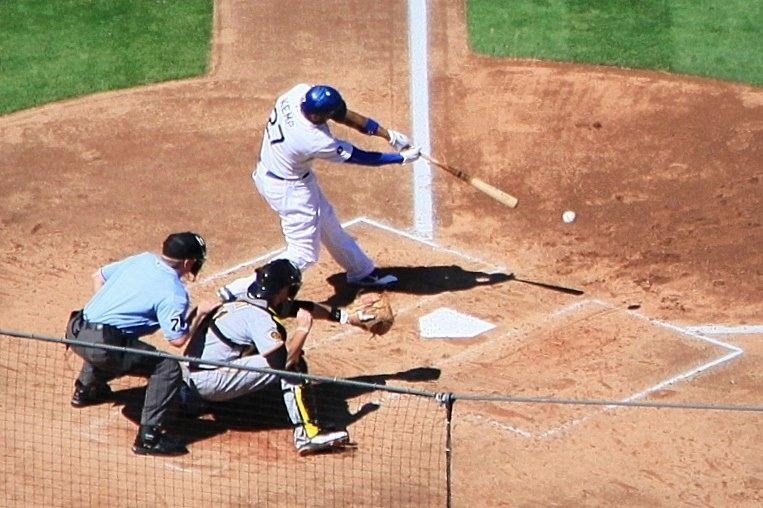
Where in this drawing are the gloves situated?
[386,128,408,151]
[399,145,421,166]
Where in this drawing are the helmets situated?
[247,259,302,300]
[162,231,206,282]
[301,85,346,120]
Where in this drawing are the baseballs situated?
[561,211,576,224]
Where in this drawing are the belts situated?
[266,170,308,181]
[79,319,103,331]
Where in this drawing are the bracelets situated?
[327,308,343,323]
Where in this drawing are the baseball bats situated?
[404,144,518,209]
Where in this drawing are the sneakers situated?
[298,430,349,455]
[71,384,111,405]
[358,268,398,285]
[132,425,188,454]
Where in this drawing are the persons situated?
[58,230,226,456]
[178,255,350,457]
[212,78,423,305]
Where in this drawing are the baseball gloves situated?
[345,291,394,336]
